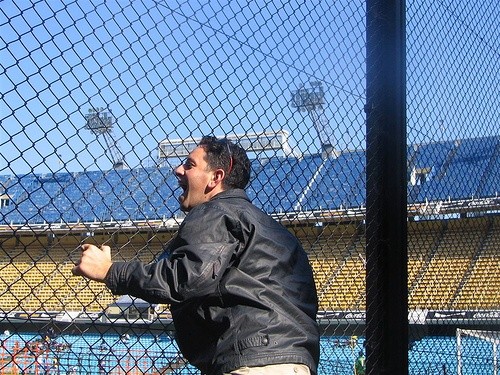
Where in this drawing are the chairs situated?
[1,133,500,375]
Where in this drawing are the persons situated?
[353,351,366,375]
[69,134,322,375]
